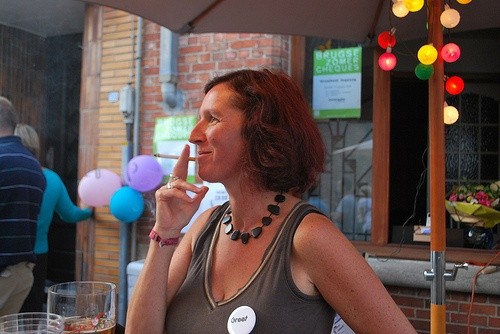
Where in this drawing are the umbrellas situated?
[87,1,500,334]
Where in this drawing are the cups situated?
[47,281,116,334]
[0,312,65,334]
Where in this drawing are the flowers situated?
[446,178,500,227]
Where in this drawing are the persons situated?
[307,178,372,240]
[125,69,419,334]
[0,96,93,332]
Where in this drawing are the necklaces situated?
[222,191,286,244]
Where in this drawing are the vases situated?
[463,224,493,250]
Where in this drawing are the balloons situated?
[78,169,121,208]
[110,186,144,222]
[125,155,164,192]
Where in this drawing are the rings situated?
[169,173,181,182]
[168,183,174,189]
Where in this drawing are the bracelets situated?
[149,228,179,248]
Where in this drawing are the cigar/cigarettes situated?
[154,154,196,161]
